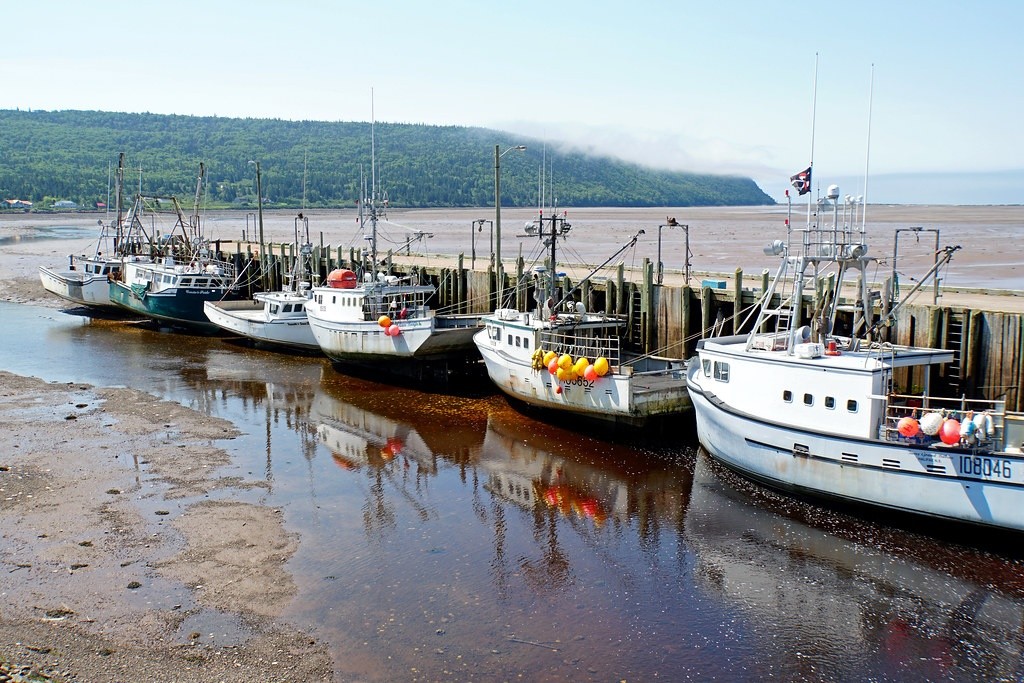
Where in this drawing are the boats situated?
[680,50,1023,535]
[470,130,695,427]
[107,160,248,335]
[304,86,486,366]
[36,154,176,313]
[203,159,324,353]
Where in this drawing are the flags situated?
[790,167,812,195]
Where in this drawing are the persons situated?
[888,378,907,418]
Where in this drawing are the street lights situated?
[494,141,528,309]
